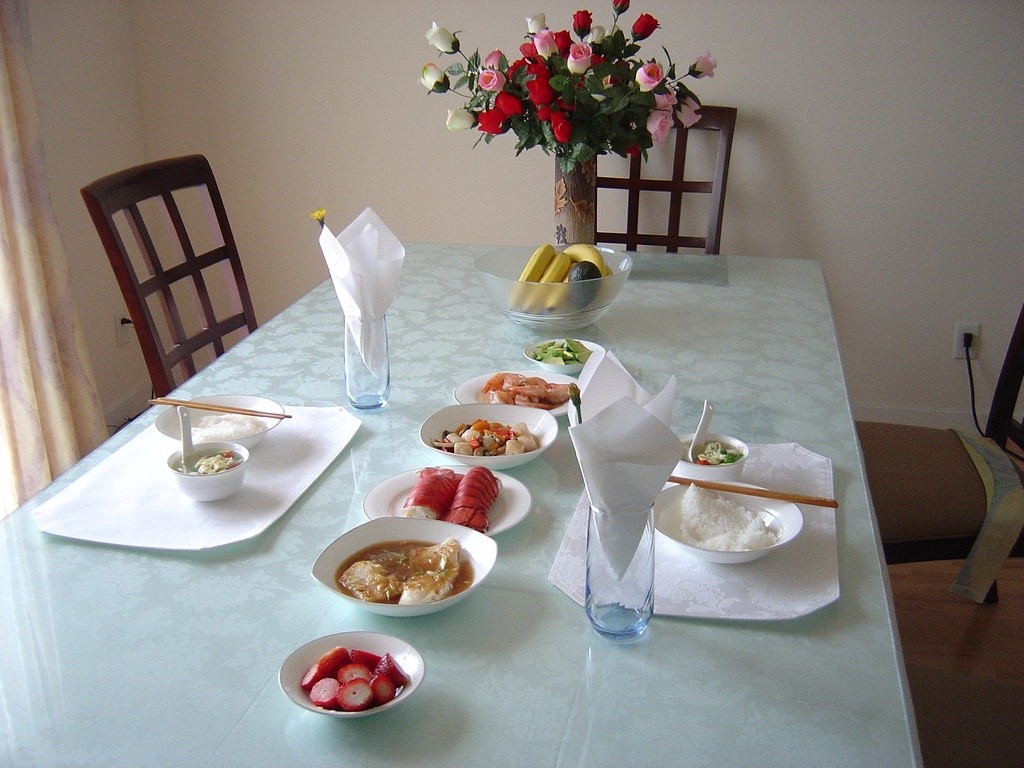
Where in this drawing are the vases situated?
[555,155,596,246]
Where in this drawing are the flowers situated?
[310,208,326,228]
[422,0,717,172]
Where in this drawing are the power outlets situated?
[954,324,981,359]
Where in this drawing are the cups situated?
[344,314,390,409]
[586,505,655,642]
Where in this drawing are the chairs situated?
[592,105,738,255]
[854,302,1024,605]
[81,154,259,398]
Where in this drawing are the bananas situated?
[508,243,614,314]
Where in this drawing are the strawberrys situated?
[302,646,408,712]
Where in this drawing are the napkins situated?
[318,206,405,367]
[567,350,684,579]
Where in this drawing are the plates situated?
[362,464,533,536]
[523,338,606,373]
[453,369,576,417]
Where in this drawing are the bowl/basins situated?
[419,403,559,470]
[167,441,250,503]
[670,432,749,481]
[311,516,498,617]
[473,242,633,332]
[278,630,426,718]
[651,481,805,564]
[155,394,285,449]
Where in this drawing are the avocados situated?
[568,261,602,308]
[532,339,595,366]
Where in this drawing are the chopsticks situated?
[666,476,839,509]
[147,397,292,420]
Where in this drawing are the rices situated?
[675,483,778,552]
[187,413,268,441]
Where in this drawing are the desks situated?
[0,245,927,767]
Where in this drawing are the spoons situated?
[688,399,715,463]
